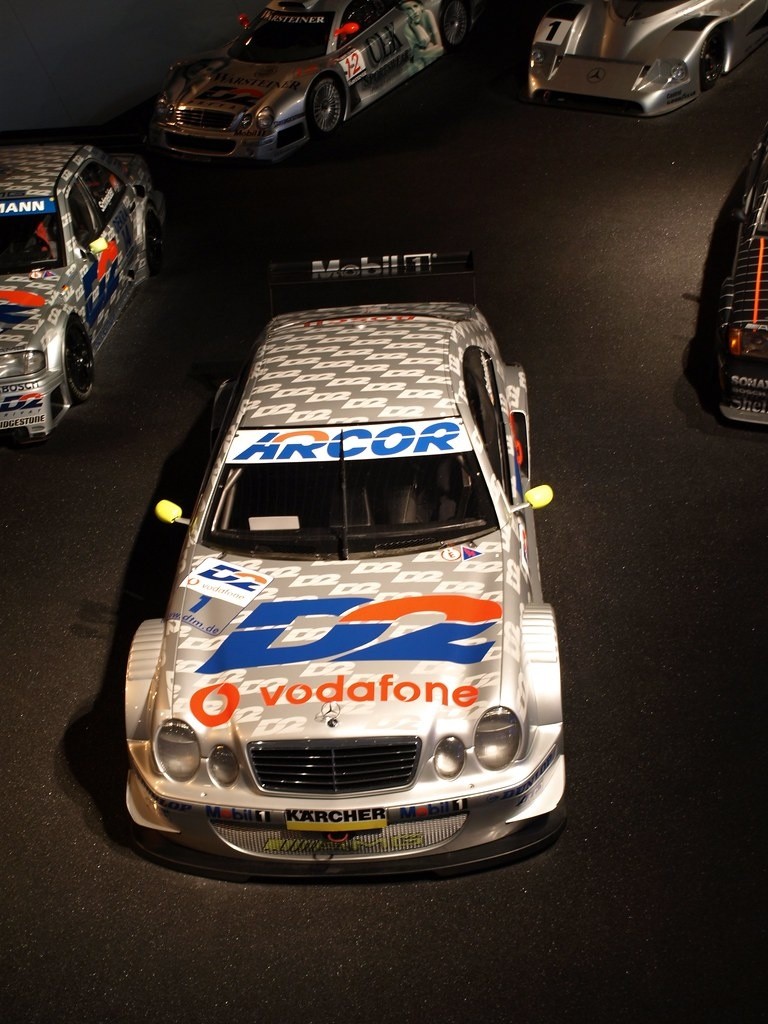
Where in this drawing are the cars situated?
[147,0,473,168]
[1,144,167,448]
[518,0,767,118]
[124,252,569,884]
[687,126,768,426]
